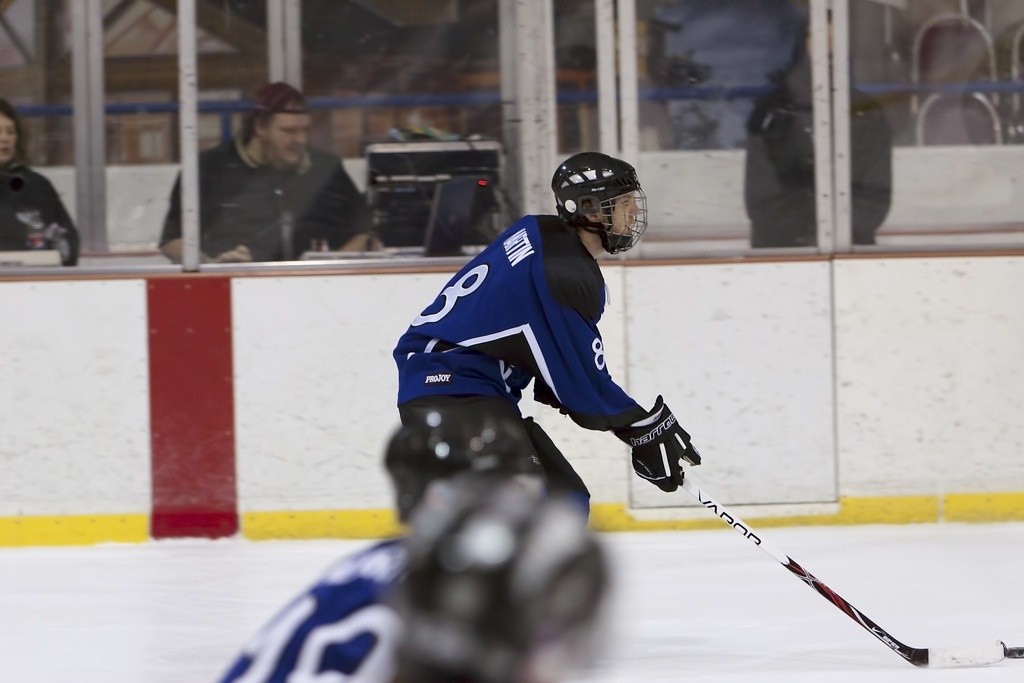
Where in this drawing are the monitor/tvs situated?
[365,140,502,255]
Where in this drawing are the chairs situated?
[911,10,1001,117]
[915,90,1003,146]
[1009,26,1024,118]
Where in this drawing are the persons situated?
[393,152,701,527]
[0,97,80,267]
[218,469,610,683]
[744,10,893,248]
[157,81,372,265]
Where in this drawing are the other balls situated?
[1006,647,1024,660]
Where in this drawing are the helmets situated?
[398,475,602,683]
[552,152,648,255]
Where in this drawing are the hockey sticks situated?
[671,465,1009,669]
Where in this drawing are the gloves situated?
[615,395,701,492]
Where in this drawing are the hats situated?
[253,82,308,114]
